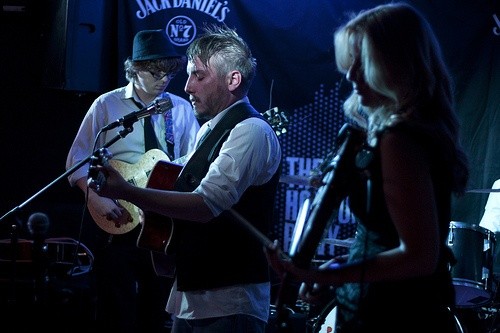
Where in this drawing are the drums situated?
[447,219,497,309]
[312,298,464,333]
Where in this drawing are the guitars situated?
[84,106,290,235]
[86,147,185,258]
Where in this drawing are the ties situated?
[129,98,159,152]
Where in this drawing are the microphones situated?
[28,213,50,246]
[102,97,173,132]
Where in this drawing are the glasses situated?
[144,70,176,80]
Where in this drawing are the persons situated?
[87,24,282,333]
[263,1,469,333]
[66,29,201,333]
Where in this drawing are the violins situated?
[270,121,366,333]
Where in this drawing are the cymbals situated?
[322,237,356,249]
[278,174,319,186]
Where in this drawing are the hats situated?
[128,29,182,62]
